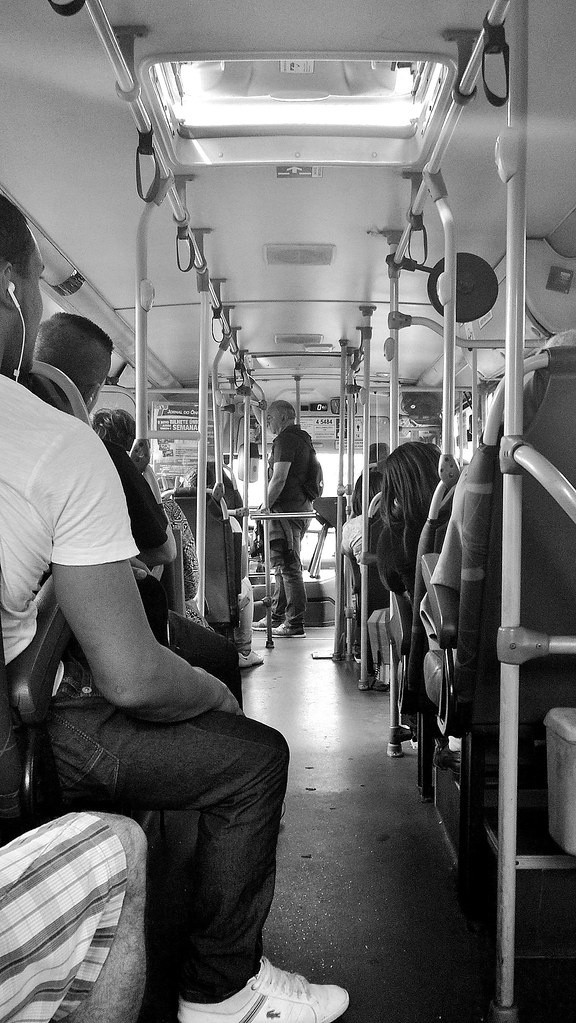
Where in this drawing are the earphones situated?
[7,281,21,309]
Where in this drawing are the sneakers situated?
[177,961,350,1023]
[239,649,264,668]
[252,616,284,631]
[270,624,306,638]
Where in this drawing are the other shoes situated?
[432,745,461,774]
[354,652,362,663]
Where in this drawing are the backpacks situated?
[279,424,324,501]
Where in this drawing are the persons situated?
[253,400,313,637]
[0,192,354,1023]
[342,331,576,624]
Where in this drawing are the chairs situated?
[0,360,576,1023]
